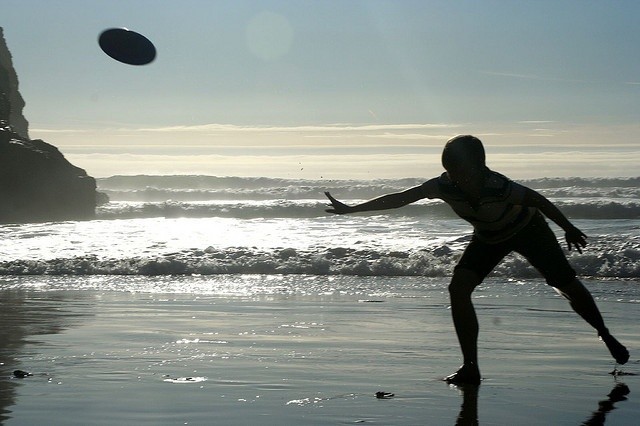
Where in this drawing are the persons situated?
[323,134,629,384]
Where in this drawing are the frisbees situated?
[98,28,155,65]
